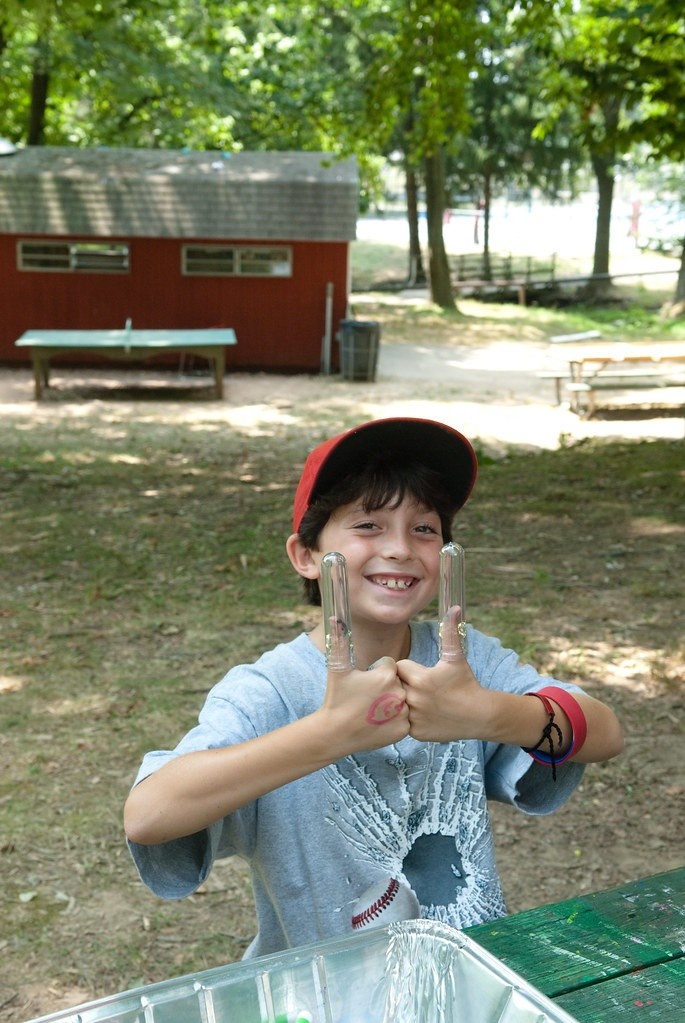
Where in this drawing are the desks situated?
[562,341,685,413]
[458,865,684,1021]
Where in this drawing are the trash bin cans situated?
[339,318,382,383]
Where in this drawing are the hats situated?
[294,417,479,533]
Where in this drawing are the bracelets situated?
[523,687,589,781]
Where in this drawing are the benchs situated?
[448,253,557,282]
[12,325,238,399]
[536,350,685,405]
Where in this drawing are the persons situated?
[123,417,628,963]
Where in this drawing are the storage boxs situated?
[20,916,578,1023]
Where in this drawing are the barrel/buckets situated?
[338,317,381,382]
